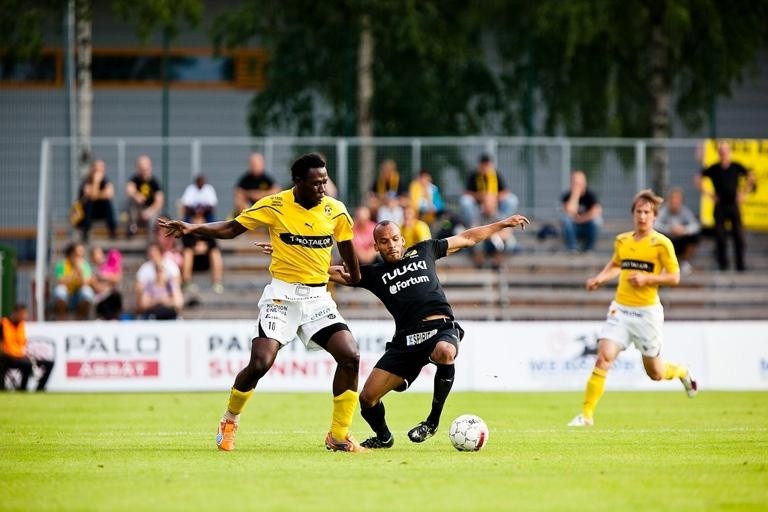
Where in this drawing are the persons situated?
[134,243,185,312]
[157,153,373,454]
[417,207,468,254]
[1,304,55,393]
[96,291,123,321]
[376,196,405,228]
[351,208,385,264]
[87,244,123,319]
[53,241,94,322]
[176,173,218,224]
[75,160,117,242]
[653,189,701,276]
[409,172,444,224]
[399,206,432,253]
[232,153,280,219]
[253,214,531,448]
[157,306,177,322]
[312,152,338,199]
[458,153,520,254]
[367,159,412,222]
[154,214,185,286]
[467,193,512,270]
[558,171,603,260]
[180,201,225,294]
[566,190,697,427]
[694,144,758,273]
[125,155,164,241]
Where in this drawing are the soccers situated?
[450,414,488,451]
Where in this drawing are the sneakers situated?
[215,285,224,294]
[187,283,197,295]
[680,261,693,273]
[360,437,394,448]
[216,419,239,451]
[408,421,438,442]
[325,431,368,453]
[681,369,698,396]
[567,415,593,426]
